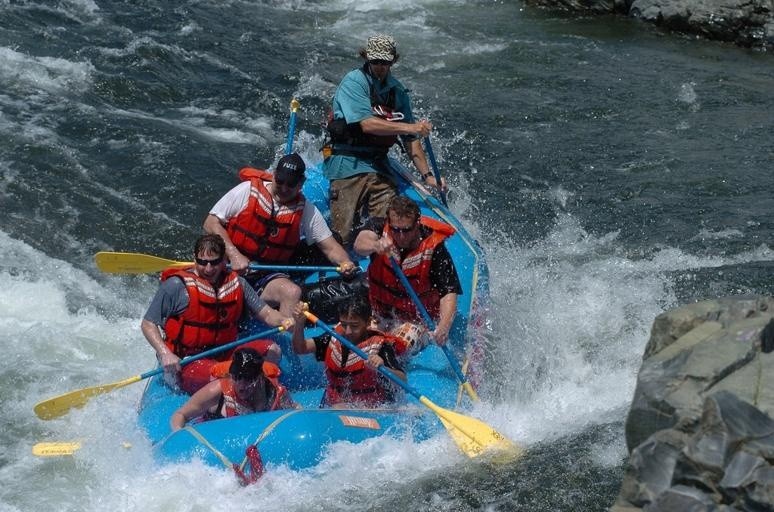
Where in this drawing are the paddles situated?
[94,252,352,273]
[301,302,511,458]
[31,430,171,458]
[33,326,286,421]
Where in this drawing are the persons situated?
[290,296,406,410]
[200,154,356,333]
[318,35,449,256]
[168,346,304,431]
[141,233,297,396]
[353,197,463,358]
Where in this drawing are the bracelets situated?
[420,171,432,182]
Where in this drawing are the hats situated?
[229,347,265,379]
[367,34,397,62]
[275,152,306,185]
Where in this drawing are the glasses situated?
[387,219,417,234]
[193,254,225,267]
[229,372,255,384]
[274,174,302,188]
[367,57,395,67]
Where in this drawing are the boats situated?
[136,135,490,489]
